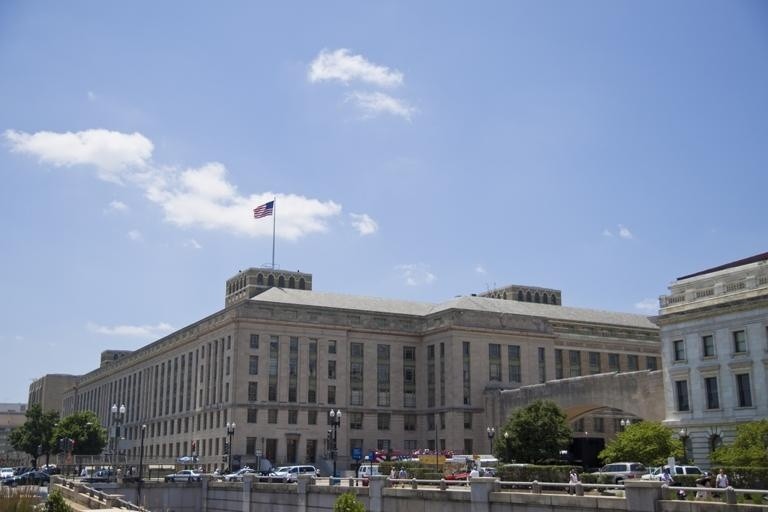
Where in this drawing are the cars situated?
[165,470,208,482]
[445,471,471,486]
[223,464,321,483]
[641,465,705,481]
[81,466,142,482]
[0,464,58,486]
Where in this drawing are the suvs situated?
[592,461,645,485]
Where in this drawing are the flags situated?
[252,201,273,218]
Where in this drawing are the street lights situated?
[679,426,689,463]
[620,418,630,431]
[112,403,125,483]
[329,407,341,476]
[226,421,236,472]
[487,427,496,453]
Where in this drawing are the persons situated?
[569,470,581,482]
[569,468,579,493]
[390,467,396,487]
[470,467,480,477]
[716,468,728,487]
[399,466,408,485]
[695,472,712,498]
[661,469,675,483]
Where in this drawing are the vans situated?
[357,465,383,478]
